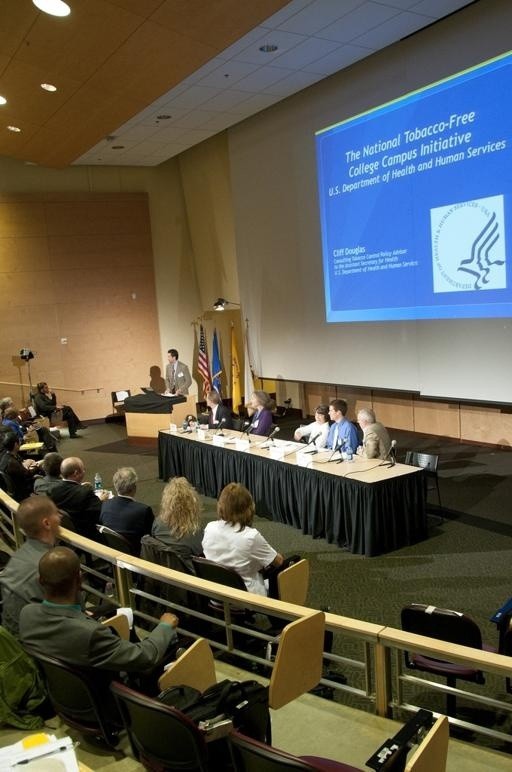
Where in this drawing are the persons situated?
[166,349,192,397]
[190,390,393,462]
[1,383,282,729]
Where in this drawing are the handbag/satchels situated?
[154,682,203,712]
[183,676,273,746]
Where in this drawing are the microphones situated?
[244,421,255,433]
[214,417,227,433]
[310,431,324,445]
[183,415,193,426]
[268,424,281,440]
[334,439,348,454]
[383,439,398,459]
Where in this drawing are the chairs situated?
[405,451,443,526]
[227,686,433,771]
[109,680,239,771]
[55,508,112,590]
[17,615,190,760]
[139,534,196,621]
[401,603,499,741]
[93,523,140,589]
[197,413,210,425]
[31,392,58,442]
[191,555,310,635]
[111,390,131,415]
[232,418,245,432]
[490,599,512,693]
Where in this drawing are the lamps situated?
[214,298,228,312]
[21,349,34,391]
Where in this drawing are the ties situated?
[331,424,339,449]
[170,364,176,391]
[208,411,214,425]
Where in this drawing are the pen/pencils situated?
[10,746,67,770]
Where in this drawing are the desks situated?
[157,424,429,558]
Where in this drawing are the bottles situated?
[94,472,103,490]
[348,445,353,460]
[196,420,200,434]
[182,418,187,430]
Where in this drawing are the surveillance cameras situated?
[20,348,35,361]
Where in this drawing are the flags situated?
[211,331,224,405]
[196,323,212,400]
[231,322,243,417]
[244,324,258,418]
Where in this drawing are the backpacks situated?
[0,618,50,732]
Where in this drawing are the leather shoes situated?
[76,423,89,430]
[70,433,83,438]
